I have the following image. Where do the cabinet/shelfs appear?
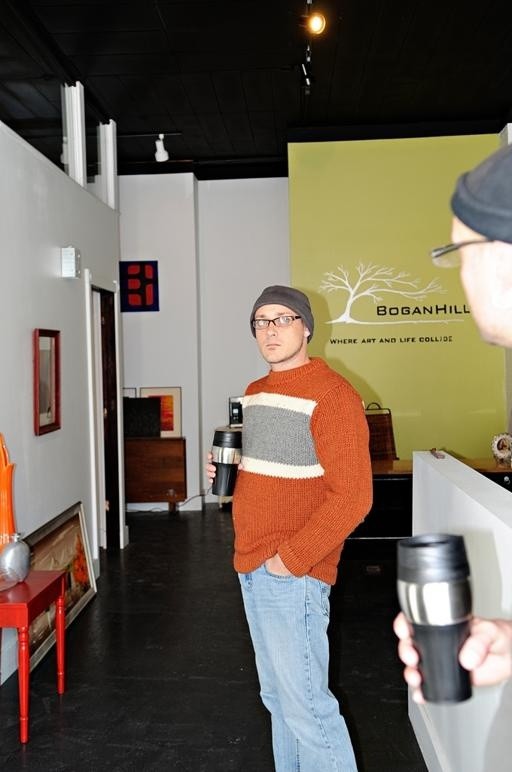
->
[123,438,187,516]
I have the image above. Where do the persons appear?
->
[391,143,511,772]
[204,283,373,772]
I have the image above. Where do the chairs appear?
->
[364,408,400,461]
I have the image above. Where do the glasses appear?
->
[431,238,494,269]
[251,316,302,329]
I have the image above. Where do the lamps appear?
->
[151,134,169,162]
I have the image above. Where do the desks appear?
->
[0,570,66,744]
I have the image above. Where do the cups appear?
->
[395,531,477,705]
[210,429,243,497]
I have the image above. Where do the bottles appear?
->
[1,532,32,583]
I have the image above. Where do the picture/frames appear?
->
[121,387,182,440]
[14,501,98,678]
[32,328,61,437]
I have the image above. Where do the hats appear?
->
[450,141,512,244]
[250,285,314,343]
